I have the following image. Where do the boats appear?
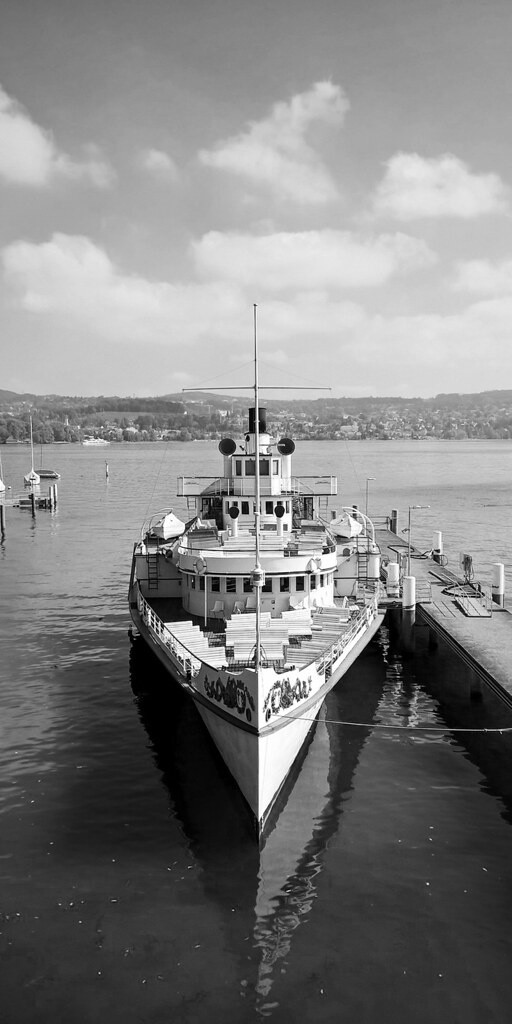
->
[329,511,362,539]
[153,512,186,541]
[129,304,388,847]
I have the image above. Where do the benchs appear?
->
[187,528,327,557]
[162,607,351,675]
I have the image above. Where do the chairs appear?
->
[209,600,226,619]
[232,600,245,614]
[314,598,324,614]
[244,595,257,613]
[288,596,303,611]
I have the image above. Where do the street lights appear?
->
[408,505,432,576]
[366,478,375,516]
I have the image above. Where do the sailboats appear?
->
[0,457,5,492]
[35,429,60,478]
[23,414,41,485]
